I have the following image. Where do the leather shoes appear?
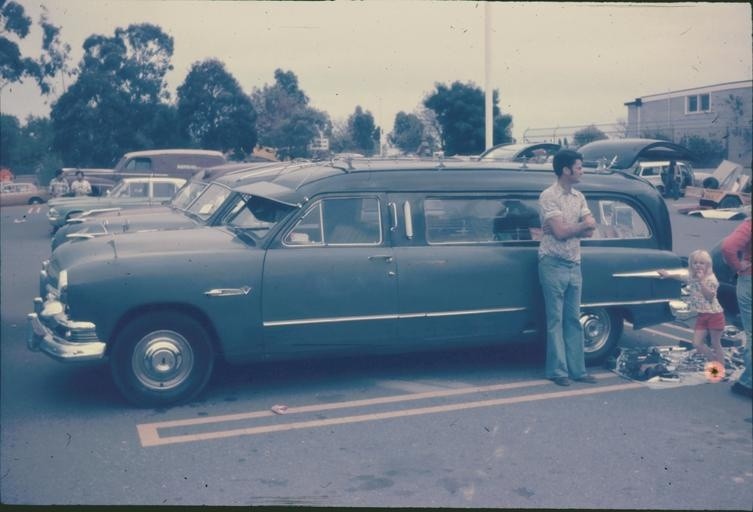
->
[576,375,598,383]
[554,377,569,386]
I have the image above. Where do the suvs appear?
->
[53,150,229,187]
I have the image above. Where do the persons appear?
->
[658,250,725,384]
[71,171,92,198]
[664,160,681,199]
[722,214,753,398]
[537,150,598,386]
[48,169,71,197]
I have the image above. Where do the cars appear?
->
[1,180,48,208]
[33,142,568,257]
[635,161,694,194]
[25,137,698,409]
